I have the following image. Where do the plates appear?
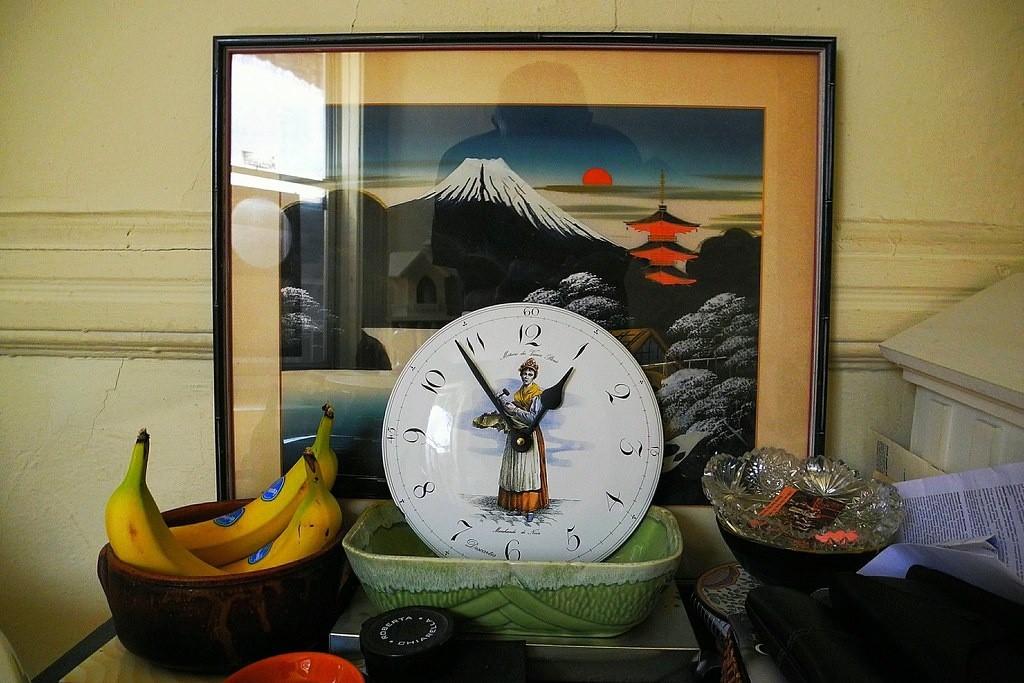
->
[225,650,365,683]
[704,448,903,550]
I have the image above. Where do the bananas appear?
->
[164,402,337,567]
[219,447,342,572]
[104,427,230,576]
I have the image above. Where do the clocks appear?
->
[381,303,665,565]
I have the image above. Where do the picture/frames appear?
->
[212,32,837,506]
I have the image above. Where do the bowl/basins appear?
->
[97,498,343,674]
[716,511,894,597]
